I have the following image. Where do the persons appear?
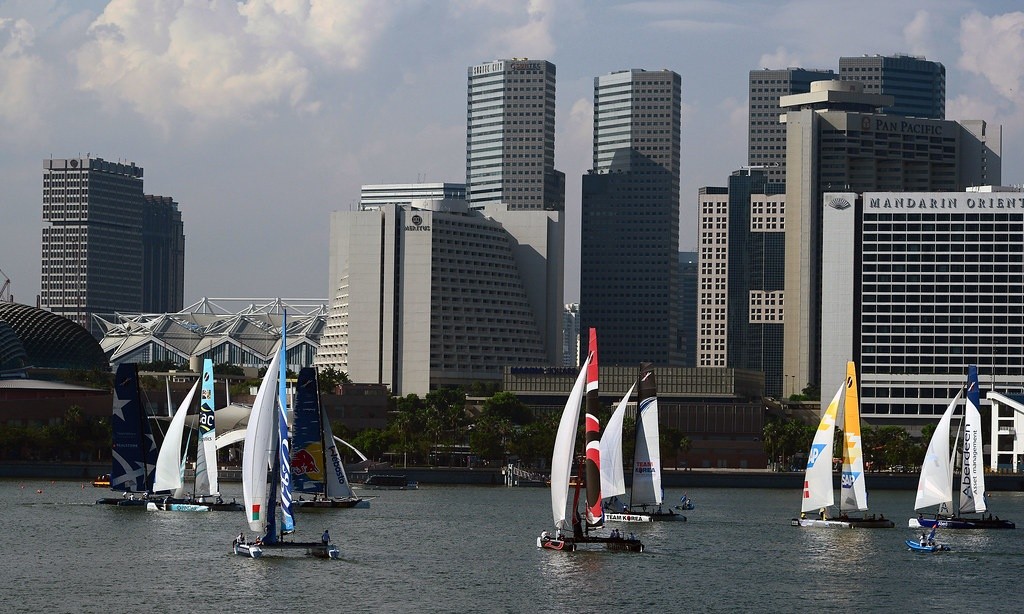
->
[611,529,634,540]
[123,491,149,499]
[541,530,550,541]
[199,495,205,503]
[871,513,884,520]
[236,533,262,545]
[686,498,691,506]
[989,513,999,520]
[603,502,608,510]
[217,496,223,504]
[920,534,927,547]
[321,530,330,546]
[657,507,673,516]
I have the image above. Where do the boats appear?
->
[361,474,420,491]
[673,502,695,511]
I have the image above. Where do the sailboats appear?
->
[536,328,646,555]
[579,361,687,523]
[232,308,341,562]
[904,514,952,553]
[813,361,896,529]
[91,352,374,513]
[908,364,1017,530]
[790,380,856,530]
